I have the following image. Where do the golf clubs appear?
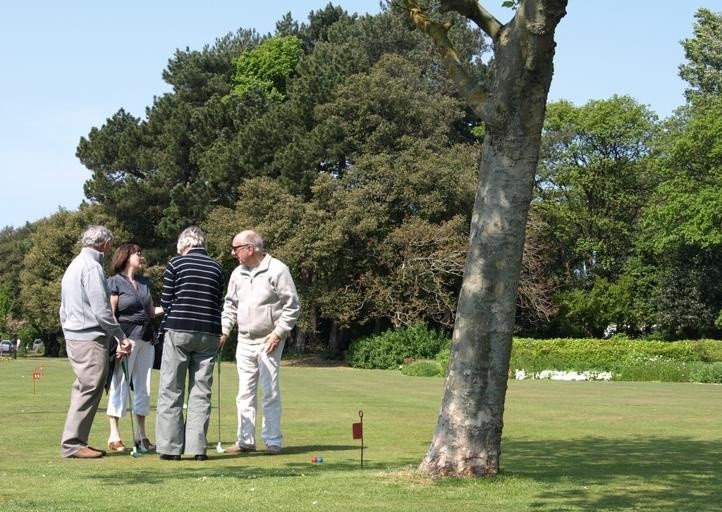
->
[121,353,149,458]
[216,351,224,454]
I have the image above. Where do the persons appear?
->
[58,224,133,458]
[217,229,302,454]
[155,224,227,463]
[99,239,164,455]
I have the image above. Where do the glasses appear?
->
[230,243,255,253]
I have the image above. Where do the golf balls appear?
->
[312,457,317,462]
[317,457,323,462]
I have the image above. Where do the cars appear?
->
[33,338,45,352]
[1,339,15,353]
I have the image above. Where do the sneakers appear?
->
[223,443,256,453]
[133,437,156,451]
[195,454,208,461]
[108,439,125,451]
[264,445,282,454]
[71,445,107,458]
[159,454,181,461]
